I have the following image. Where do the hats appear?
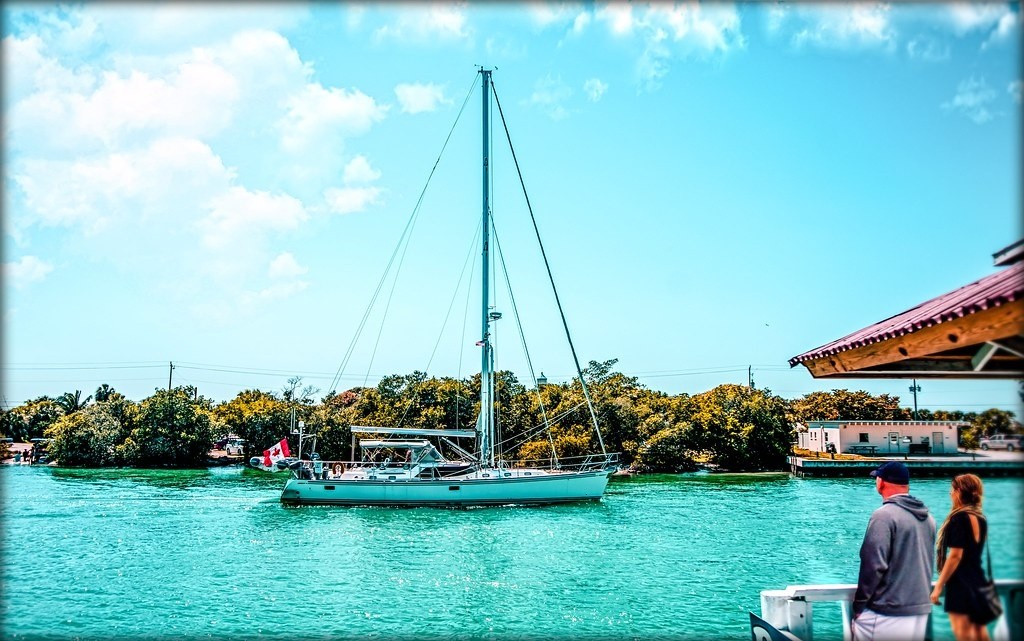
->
[870,461,909,484]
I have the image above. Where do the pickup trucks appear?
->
[980,434,1021,451]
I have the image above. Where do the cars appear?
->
[226,439,255,456]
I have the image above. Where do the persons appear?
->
[620,460,646,474]
[929,473,1003,641]
[15,444,36,461]
[303,458,329,480]
[825,442,835,453]
[851,461,938,641]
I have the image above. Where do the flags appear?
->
[263,438,290,466]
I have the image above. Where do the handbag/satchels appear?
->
[967,581,1004,625]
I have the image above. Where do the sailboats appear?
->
[279,63,620,509]
